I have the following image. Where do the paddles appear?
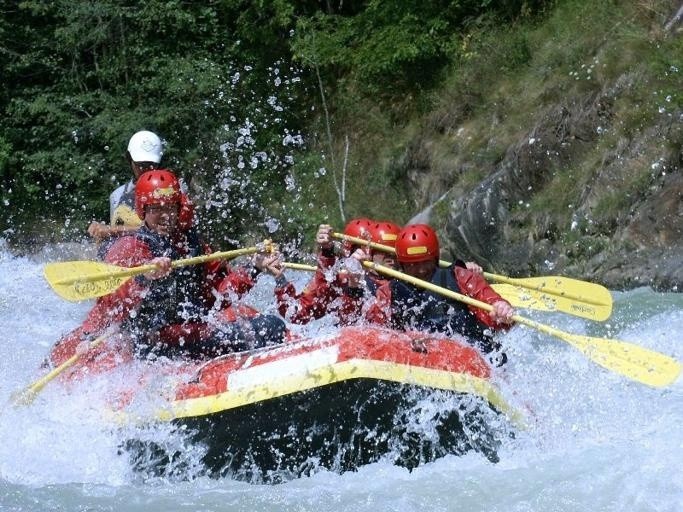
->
[331,230,615,322]
[45,239,274,298]
[360,260,682,390]
[281,259,557,311]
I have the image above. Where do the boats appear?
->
[67,327,518,484]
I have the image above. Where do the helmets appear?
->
[373,217,400,253]
[396,223,442,263]
[134,170,182,221]
[342,219,382,252]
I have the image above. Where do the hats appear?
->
[126,129,164,164]
